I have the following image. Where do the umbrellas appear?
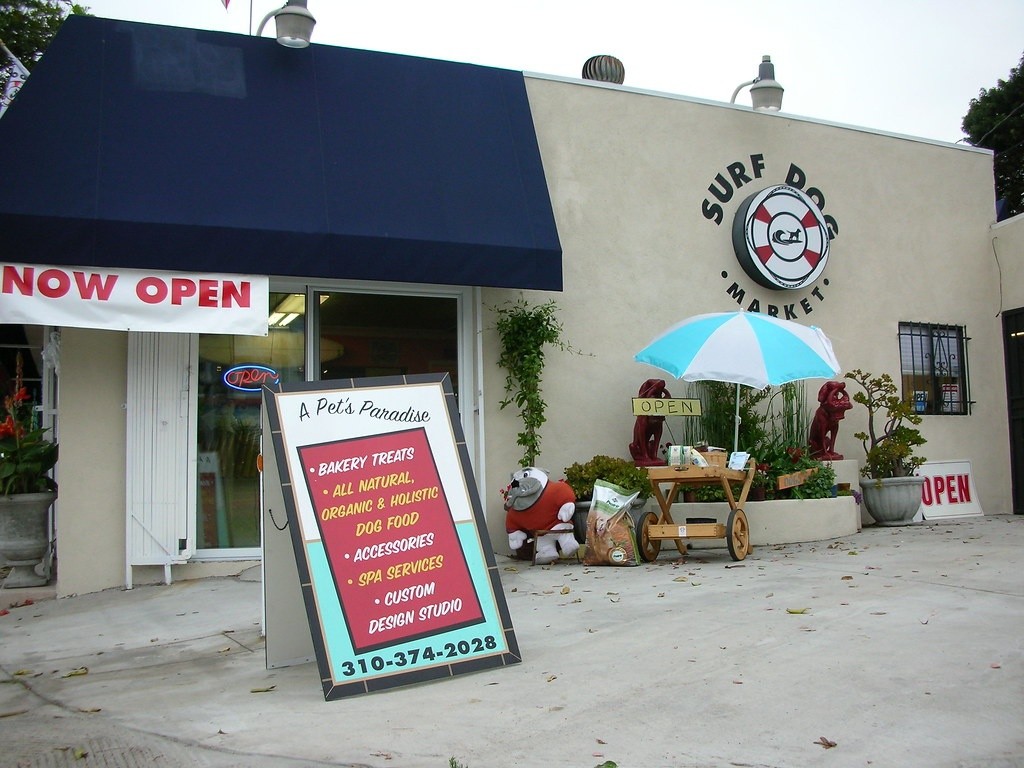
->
[633,307,841,451]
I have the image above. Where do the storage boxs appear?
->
[668,444,693,466]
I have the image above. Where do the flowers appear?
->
[0,387,58,497]
[677,381,863,505]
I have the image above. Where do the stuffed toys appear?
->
[505,467,580,562]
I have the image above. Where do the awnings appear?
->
[0,14,564,293]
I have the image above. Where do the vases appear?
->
[0,492,57,560]
[830,485,837,498]
[685,491,697,502]
[753,487,765,500]
[838,482,850,492]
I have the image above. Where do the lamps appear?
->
[268,292,329,327]
[257,0,317,48]
[730,55,784,112]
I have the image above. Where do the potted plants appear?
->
[563,455,656,546]
[845,369,928,526]
[474,292,598,559]
